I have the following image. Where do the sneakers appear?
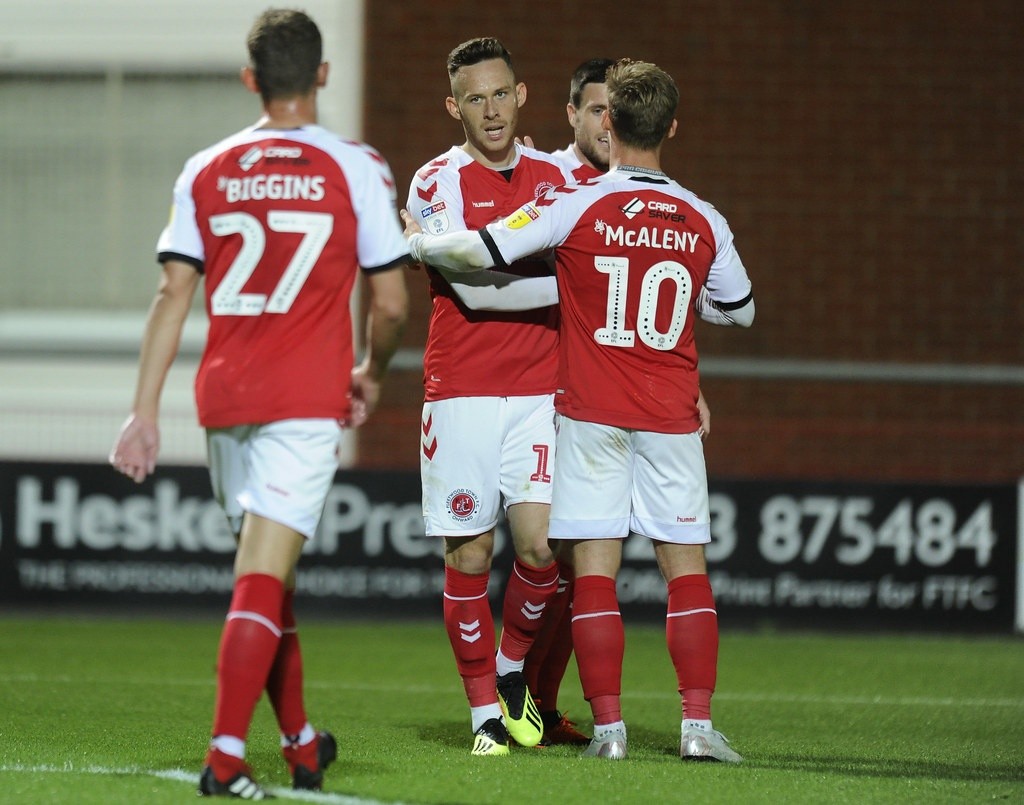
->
[282,731,336,792]
[200,766,278,801]
[496,650,544,746]
[680,721,744,763]
[470,715,510,756]
[581,720,628,760]
[543,711,594,747]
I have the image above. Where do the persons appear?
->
[399,58,756,762]
[407,36,585,754]
[512,60,624,746]
[109,8,413,800]
